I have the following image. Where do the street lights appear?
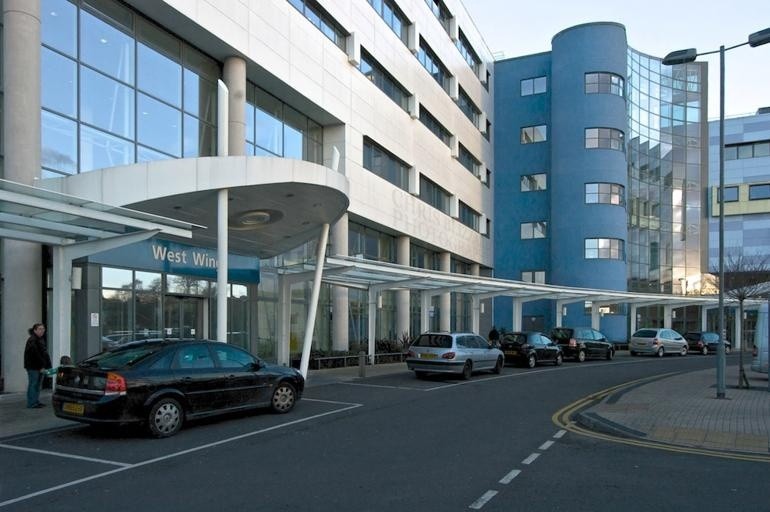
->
[662,27,770,399]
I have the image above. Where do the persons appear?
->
[499,331,505,344]
[23,323,53,408]
[39,356,75,375]
[551,328,567,344]
[489,325,500,347]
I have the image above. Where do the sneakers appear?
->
[33,403,46,408]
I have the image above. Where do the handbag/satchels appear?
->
[39,376,52,390]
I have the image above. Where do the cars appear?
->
[550,326,616,363]
[53,337,303,440]
[405,329,505,380]
[684,332,731,355]
[628,327,689,358]
[499,332,564,368]
